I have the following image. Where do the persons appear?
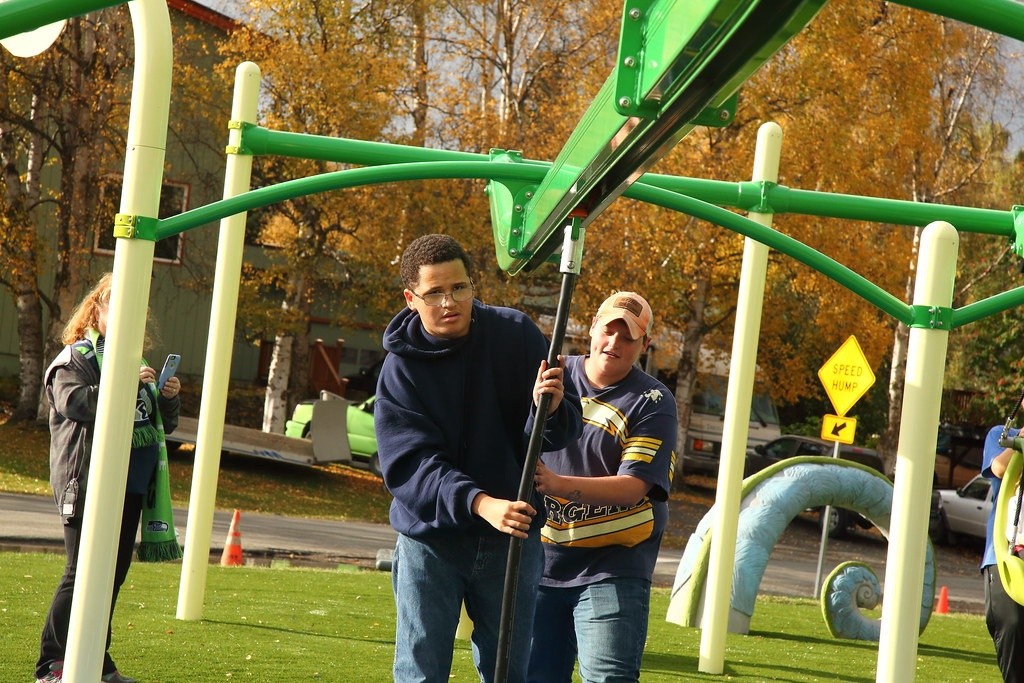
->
[979,387,1024,683]
[533,292,679,683]
[35,270,181,683]
[374,234,584,683]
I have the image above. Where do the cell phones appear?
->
[156,354,181,389]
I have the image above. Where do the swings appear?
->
[993,388,1023,608]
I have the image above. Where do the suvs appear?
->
[662,369,783,475]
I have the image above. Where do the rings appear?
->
[172,389,175,394]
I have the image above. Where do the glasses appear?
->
[407,273,475,306]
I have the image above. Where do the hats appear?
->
[597,292,653,340]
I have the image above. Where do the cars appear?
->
[939,471,997,538]
[285,397,382,480]
[744,435,887,539]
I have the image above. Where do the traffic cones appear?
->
[934,585,951,617]
[219,507,245,568]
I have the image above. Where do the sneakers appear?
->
[35,662,61,683]
[102,672,132,683]
